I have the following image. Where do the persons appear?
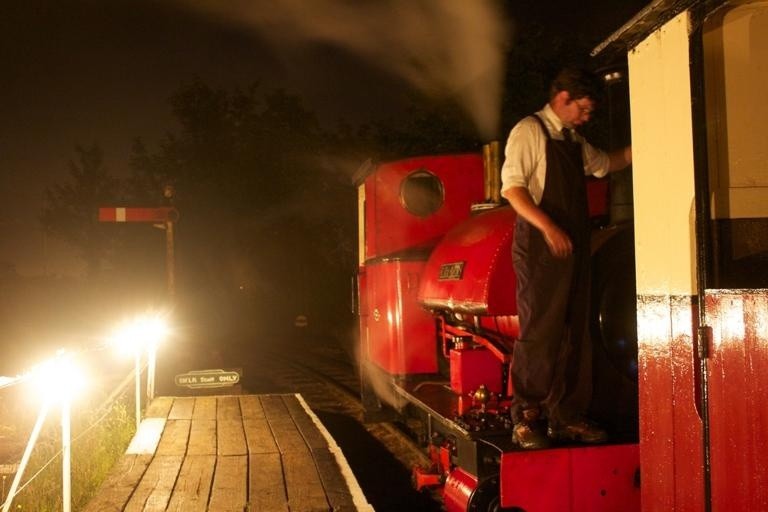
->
[501,67,632,452]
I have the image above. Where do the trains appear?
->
[353,0,768,511]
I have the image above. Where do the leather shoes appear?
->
[512,423,547,449]
[547,422,607,442]
